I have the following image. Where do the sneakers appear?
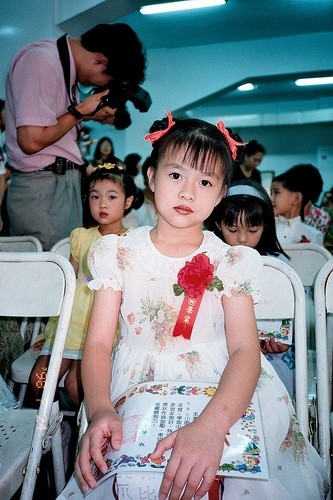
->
[61,421,71,473]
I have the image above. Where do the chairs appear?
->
[0,236,333,500]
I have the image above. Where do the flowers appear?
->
[172,251,224,340]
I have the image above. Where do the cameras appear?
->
[94,79,152,131]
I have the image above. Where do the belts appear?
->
[6,161,79,173]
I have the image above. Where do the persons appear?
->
[0,99,333,500]
[6,22,148,409]
